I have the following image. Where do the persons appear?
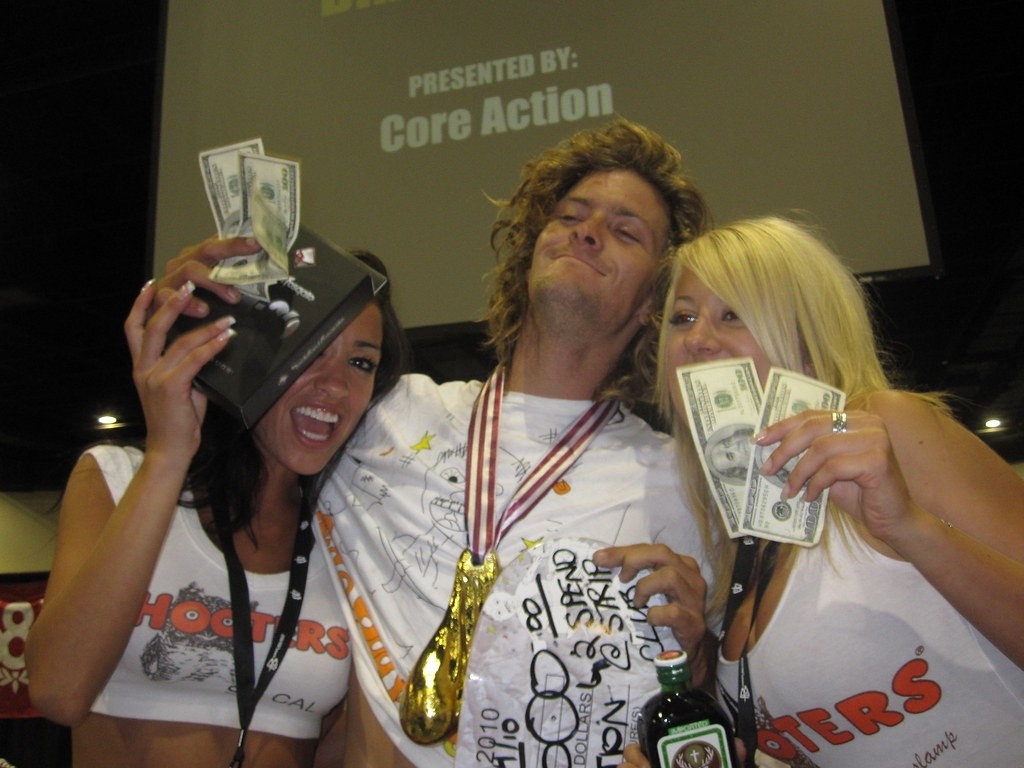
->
[24,124,720,768]
[620,218,1024,768]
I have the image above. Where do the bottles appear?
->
[637,649,742,768]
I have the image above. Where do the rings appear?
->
[830,411,850,437]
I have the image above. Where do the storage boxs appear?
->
[160,224,388,431]
